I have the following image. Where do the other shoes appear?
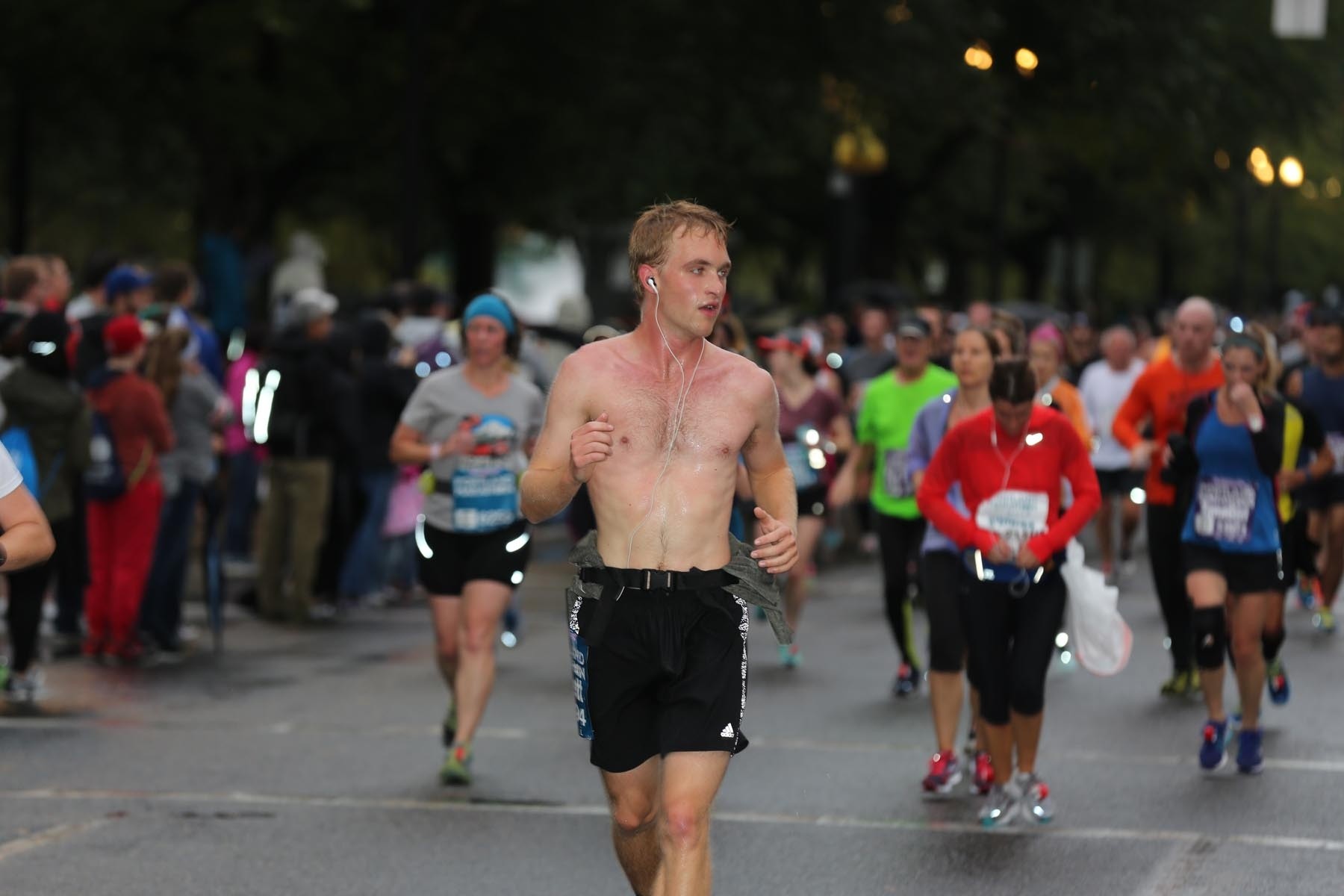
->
[1318,606,1335,635]
[1294,574,1322,609]
[1160,668,1201,694]
[1198,714,1232,771]
[1267,659,1290,705]
[778,641,802,666]
[85,639,146,665]
[1236,726,1266,774]
[978,772,1054,827]
[440,743,472,786]
[923,750,963,793]
[893,662,919,696]
[967,749,996,795]
[442,704,457,744]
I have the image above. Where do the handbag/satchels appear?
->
[1058,537,1131,677]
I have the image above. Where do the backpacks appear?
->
[85,384,153,500]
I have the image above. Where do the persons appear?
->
[518,201,799,896]
[706,294,1344,827]
[0,249,592,786]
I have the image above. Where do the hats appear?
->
[896,313,932,337]
[362,322,402,349]
[756,329,821,357]
[104,264,157,302]
[285,286,339,322]
[105,313,159,358]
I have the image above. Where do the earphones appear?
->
[649,277,660,294]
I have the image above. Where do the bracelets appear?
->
[430,443,442,459]
